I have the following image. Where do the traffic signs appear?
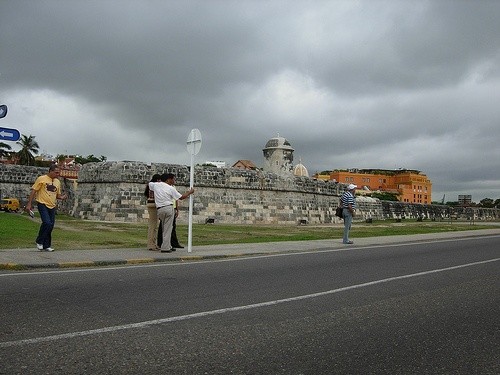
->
[1,127,20,142]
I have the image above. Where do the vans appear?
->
[0,198,20,213]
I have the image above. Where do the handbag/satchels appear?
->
[335,207,343,218]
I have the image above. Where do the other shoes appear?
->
[45,247,55,252]
[344,240,353,244]
[161,249,172,253]
[173,245,184,249]
[36,242,43,250]
[171,249,176,251]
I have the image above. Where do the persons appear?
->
[27,165,68,252]
[143,172,195,254]
[339,183,357,244]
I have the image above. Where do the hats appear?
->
[348,184,357,191]
